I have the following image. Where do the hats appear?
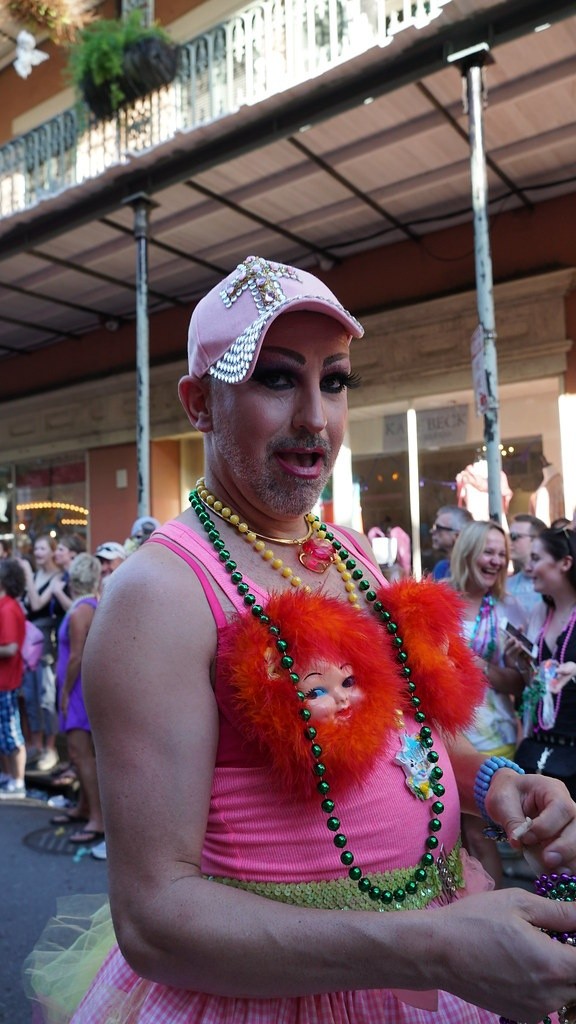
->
[96,542,125,560]
[187,255,365,387]
[131,516,161,536]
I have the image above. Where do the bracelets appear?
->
[476,755,526,842]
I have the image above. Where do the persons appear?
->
[36,255,575,1024]
[0,517,157,861]
[431,504,575,802]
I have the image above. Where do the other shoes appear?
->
[90,841,108,858]
[0,782,25,801]
[36,748,59,770]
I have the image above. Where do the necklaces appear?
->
[196,477,360,612]
[198,486,336,573]
[472,597,498,665]
[187,468,446,902]
[538,608,575,729]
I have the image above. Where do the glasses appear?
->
[433,524,455,532]
[509,532,531,541]
[556,524,572,546]
[96,545,120,552]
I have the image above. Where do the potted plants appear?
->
[62,9,179,120]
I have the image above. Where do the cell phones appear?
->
[498,617,539,659]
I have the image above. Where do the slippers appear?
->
[49,813,87,825]
[69,830,104,844]
